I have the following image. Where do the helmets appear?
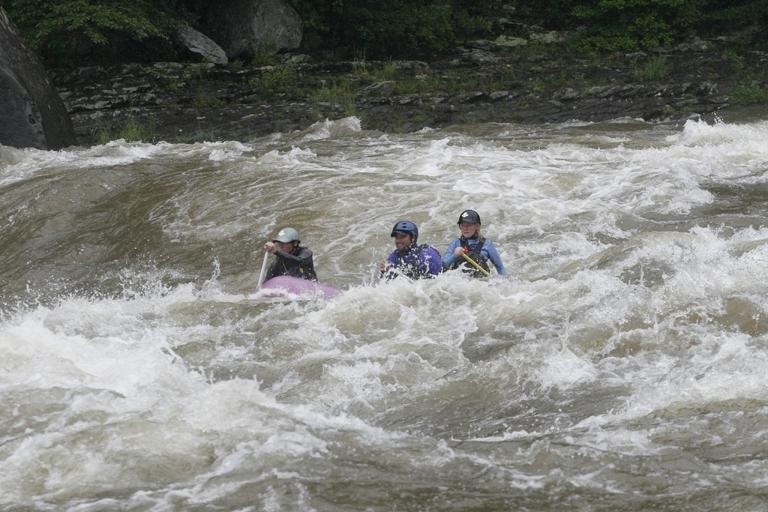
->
[273,227,300,245]
[457,210,481,224]
[391,220,418,242]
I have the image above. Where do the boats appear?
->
[264,275,346,302]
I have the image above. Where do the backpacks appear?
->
[457,236,491,279]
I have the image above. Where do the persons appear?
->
[262,226,317,286]
[442,209,508,282]
[377,219,442,279]
